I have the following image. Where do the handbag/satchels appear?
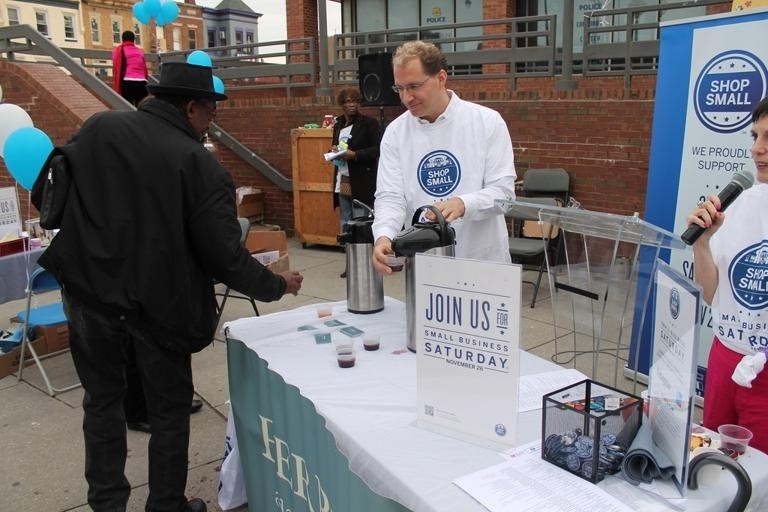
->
[40,155,72,229]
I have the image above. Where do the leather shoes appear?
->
[127,420,151,433]
[187,498,207,512]
[189,400,202,412]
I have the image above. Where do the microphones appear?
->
[680,170,755,246]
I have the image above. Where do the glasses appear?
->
[201,104,217,118]
[391,77,430,93]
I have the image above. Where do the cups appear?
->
[336,349,355,368]
[387,254,405,272]
[361,332,379,351]
[324,114,333,129]
[716,424,753,462]
[316,304,333,320]
[334,339,353,356]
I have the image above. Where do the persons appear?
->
[35,58,305,510]
[327,85,382,277]
[689,98,767,452]
[368,40,517,278]
[112,30,152,107]
[122,95,204,436]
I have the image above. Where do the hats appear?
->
[146,62,227,101]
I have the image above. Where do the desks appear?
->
[223,295,768,512]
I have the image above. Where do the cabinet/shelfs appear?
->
[289,126,346,251]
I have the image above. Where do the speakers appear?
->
[358,52,403,107]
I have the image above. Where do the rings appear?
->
[696,200,704,209]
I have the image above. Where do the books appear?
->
[320,150,347,162]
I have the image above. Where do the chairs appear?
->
[214,218,260,335]
[505,167,572,308]
[16,265,82,397]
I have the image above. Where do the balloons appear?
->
[209,75,225,95]
[186,51,213,68]
[3,126,55,190]
[133,2,151,27]
[144,0,162,18]
[0,104,35,158]
[155,0,180,27]
